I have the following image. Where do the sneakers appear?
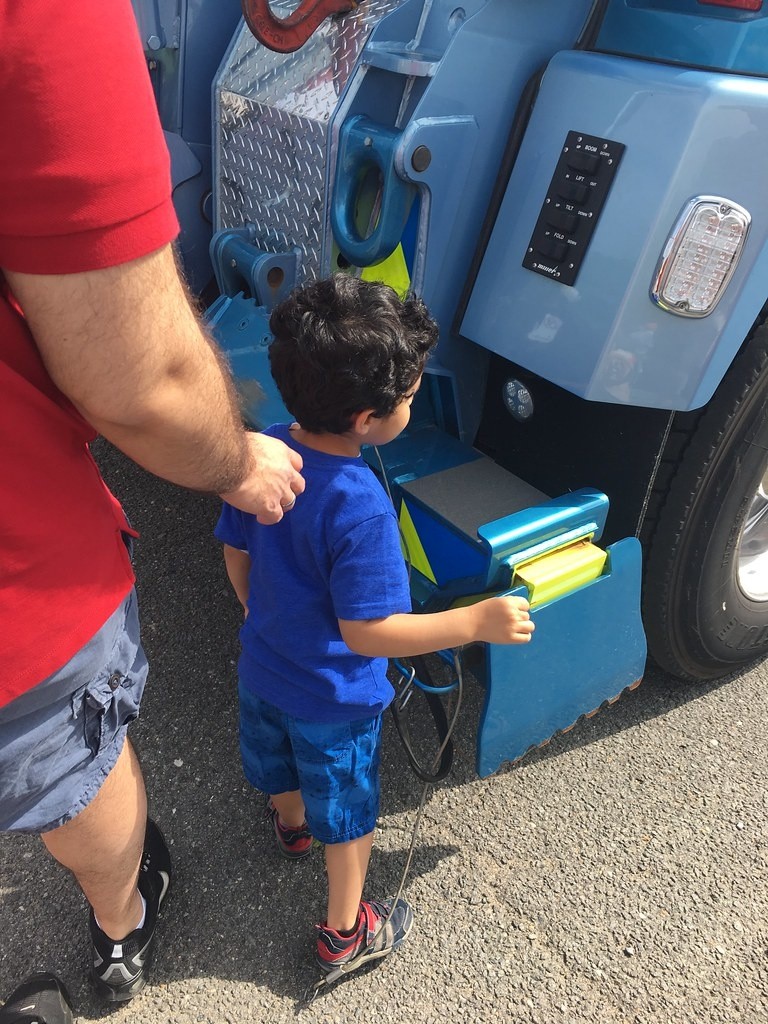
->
[89,816,171,1001]
[315,899,414,972]
[263,797,313,859]
[0,970,73,1024]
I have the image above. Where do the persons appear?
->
[215,274,535,995]
[0,3,310,1004]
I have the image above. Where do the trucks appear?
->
[125,0,768,780]
